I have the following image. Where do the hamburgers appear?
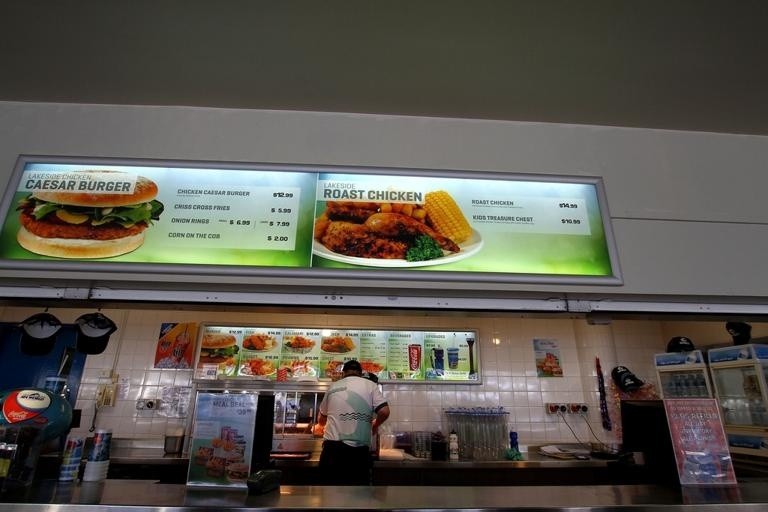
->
[226,463,249,483]
[225,453,243,470]
[552,368,562,377]
[200,334,239,366]
[16,170,164,259]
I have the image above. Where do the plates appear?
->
[243,341,277,352]
[321,347,356,355]
[286,367,317,379]
[240,364,276,377]
[314,219,484,268]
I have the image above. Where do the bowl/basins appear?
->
[285,345,313,354]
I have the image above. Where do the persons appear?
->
[317,359,390,486]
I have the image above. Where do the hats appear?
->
[342,360,362,371]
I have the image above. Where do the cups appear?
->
[45,376,66,395]
[447,348,459,369]
[171,334,191,364]
[408,345,421,371]
[221,426,245,455]
[57,429,113,482]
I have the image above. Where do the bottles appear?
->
[434,345,444,370]
[509,428,519,451]
[664,374,704,398]
[449,430,459,461]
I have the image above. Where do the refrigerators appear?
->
[653,342,768,459]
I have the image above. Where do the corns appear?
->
[423,191,472,245]
